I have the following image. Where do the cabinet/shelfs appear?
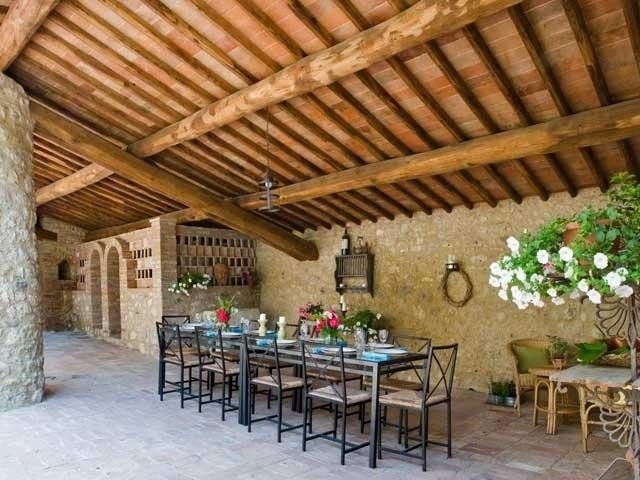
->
[334,253,375,298]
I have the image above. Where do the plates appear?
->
[374,349,408,355]
[323,347,357,353]
[367,343,395,349]
[221,333,241,336]
[254,329,275,334]
[276,340,298,344]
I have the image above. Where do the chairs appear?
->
[360,335,432,444]
[156,321,213,408]
[248,323,298,409]
[194,325,255,421]
[506,337,568,418]
[299,339,373,465]
[161,315,209,394]
[243,333,313,443]
[305,369,362,429]
[377,343,458,473]
[210,320,260,391]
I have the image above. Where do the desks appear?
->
[549,363,640,454]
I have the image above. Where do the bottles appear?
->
[341,228,350,256]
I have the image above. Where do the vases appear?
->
[353,327,368,360]
[562,219,622,264]
[175,288,204,323]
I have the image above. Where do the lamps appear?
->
[257,169,280,213]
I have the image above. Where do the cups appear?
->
[379,330,388,349]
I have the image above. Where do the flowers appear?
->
[167,270,211,296]
[337,309,381,339]
[488,171,639,310]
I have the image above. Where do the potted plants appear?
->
[504,380,517,407]
[545,334,569,369]
